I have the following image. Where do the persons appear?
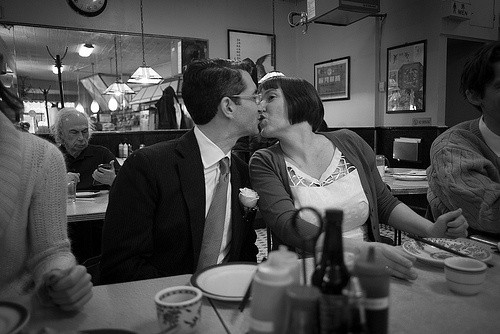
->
[95,59,261,285]
[426,42,500,232]
[51,107,122,264]
[249,76,468,279]
[0,36,94,334]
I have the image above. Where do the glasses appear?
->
[231,91,263,105]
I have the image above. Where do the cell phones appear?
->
[93,164,112,185]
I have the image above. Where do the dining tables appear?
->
[14,237,500,334]
[381,166,429,244]
[64,190,109,221]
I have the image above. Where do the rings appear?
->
[386,264,390,270]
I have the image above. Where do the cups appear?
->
[442,256,487,296]
[376,155,385,178]
[64,176,77,203]
[155,286,203,334]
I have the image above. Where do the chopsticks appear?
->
[405,233,495,269]
[238,257,269,311]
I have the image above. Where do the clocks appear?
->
[66,0,107,17]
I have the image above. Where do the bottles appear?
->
[118,143,132,158]
[249,207,391,334]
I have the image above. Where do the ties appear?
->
[194,158,230,273]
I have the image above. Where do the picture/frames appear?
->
[386,40,425,113]
[313,56,351,101]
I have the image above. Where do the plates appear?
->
[393,172,428,181]
[189,261,264,302]
[401,238,492,267]
[76,191,100,199]
[0,301,31,334]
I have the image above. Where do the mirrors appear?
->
[0,19,209,137]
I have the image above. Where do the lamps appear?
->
[127,0,163,85]
[50,32,133,113]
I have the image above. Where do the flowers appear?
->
[239,186,260,222]
